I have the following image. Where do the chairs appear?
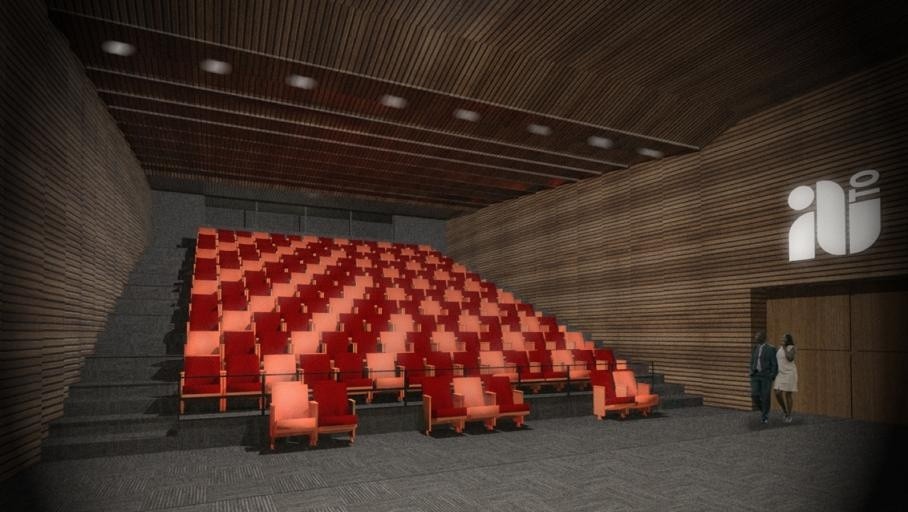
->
[180,224,659,452]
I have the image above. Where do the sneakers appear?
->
[761,416,768,423]
[784,415,792,424]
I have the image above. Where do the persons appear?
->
[772,332,799,426]
[746,330,778,423]
